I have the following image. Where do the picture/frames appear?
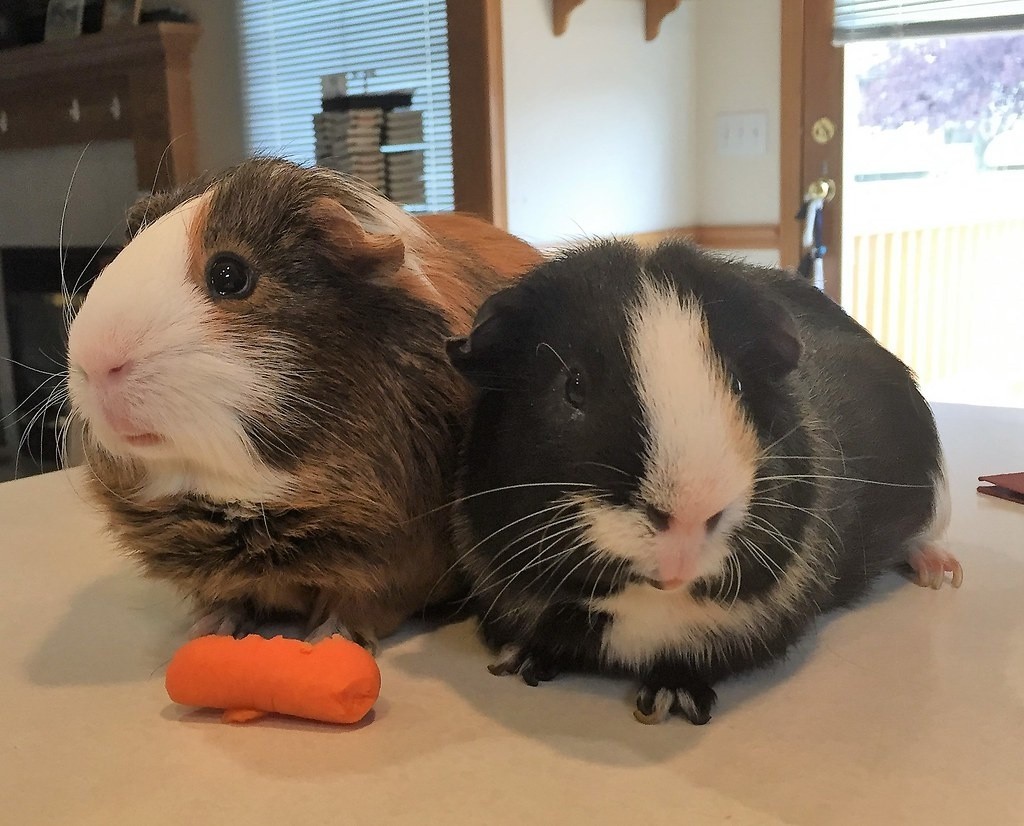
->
[101,1,141,30]
[43,0,85,42]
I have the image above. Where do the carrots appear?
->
[166,633,381,723]
